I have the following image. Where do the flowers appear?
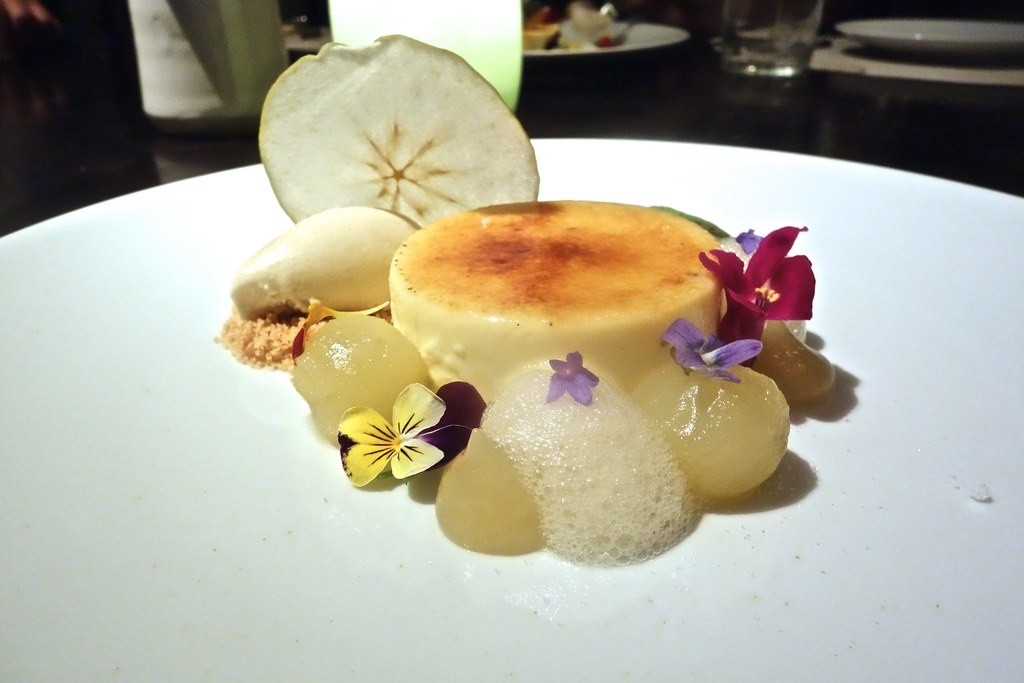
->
[699,225,815,350]
[660,319,764,382]
[337,381,486,488]
[546,352,599,405]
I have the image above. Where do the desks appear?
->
[0,0,1024,238]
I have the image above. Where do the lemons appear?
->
[259,34,538,231]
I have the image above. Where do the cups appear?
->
[127,0,288,132]
[327,0,523,120]
[720,0,827,78]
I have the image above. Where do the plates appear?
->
[282,26,331,52]
[523,23,689,59]
[837,19,1024,56]
[0,138,1024,683]
